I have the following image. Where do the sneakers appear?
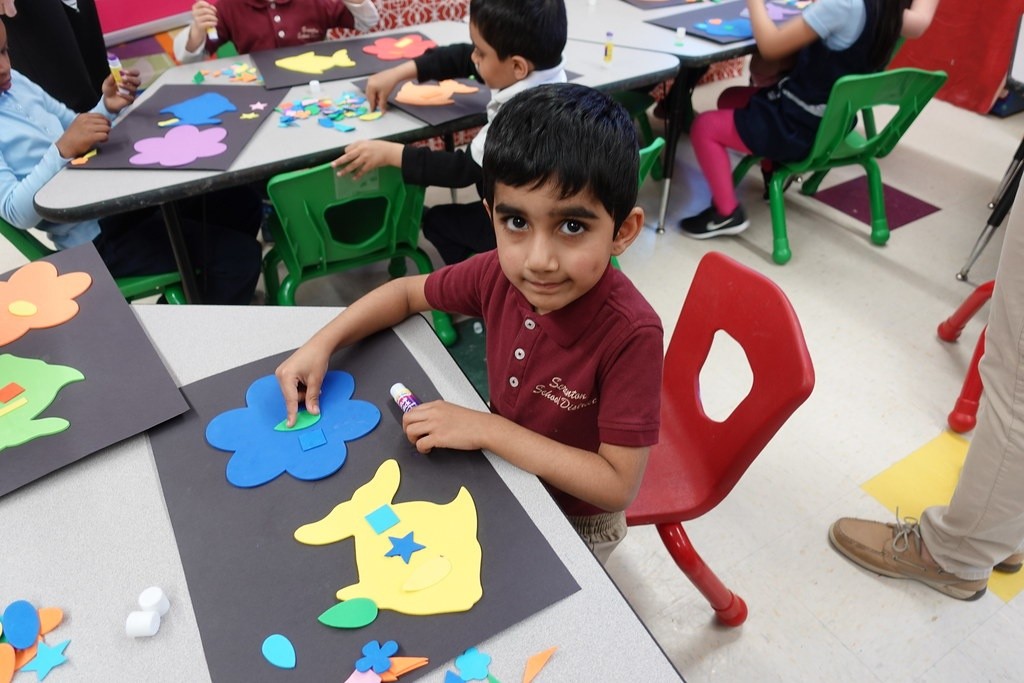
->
[678,206,750,239]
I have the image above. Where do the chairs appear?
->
[731,67,949,263]
[0,218,198,304]
[624,251,814,627]
[609,89,664,271]
[261,156,459,348]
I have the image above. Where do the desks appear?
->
[33,19,680,305]
[2,305,687,683]
[461,1,823,231]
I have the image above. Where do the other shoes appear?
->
[829,518,987,601]
[993,552,1024,574]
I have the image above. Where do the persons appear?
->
[0,18,263,304]
[171,0,379,67]
[272,82,665,577]
[828,167,1023,604]
[651,54,717,140]
[677,0,941,238]
[330,0,571,270]
[0,0,113,113]
